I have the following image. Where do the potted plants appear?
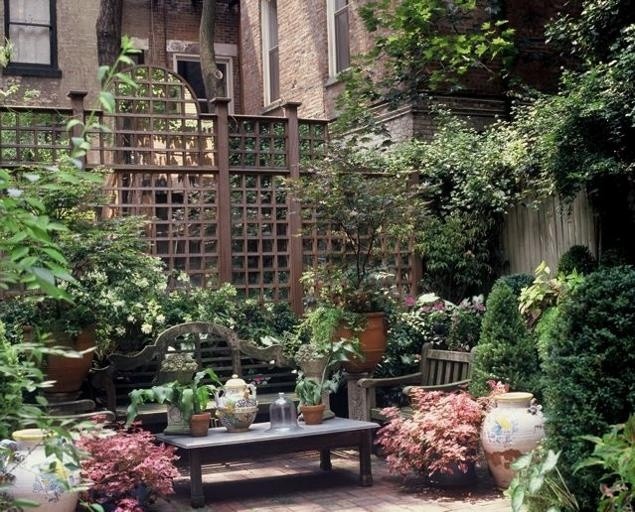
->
[294,338,366,424]
[274,136,441,371]
[124,366,227,437]
[0,149,169,394]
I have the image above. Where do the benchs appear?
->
[89,321,330,437]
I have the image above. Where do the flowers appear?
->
[373,380,511,484]
[71,413,181,511]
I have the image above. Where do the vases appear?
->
[1,428,81,512]
[423,445,470,489]
[480,392,547,492]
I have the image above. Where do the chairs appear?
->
[356,343,472,490]
